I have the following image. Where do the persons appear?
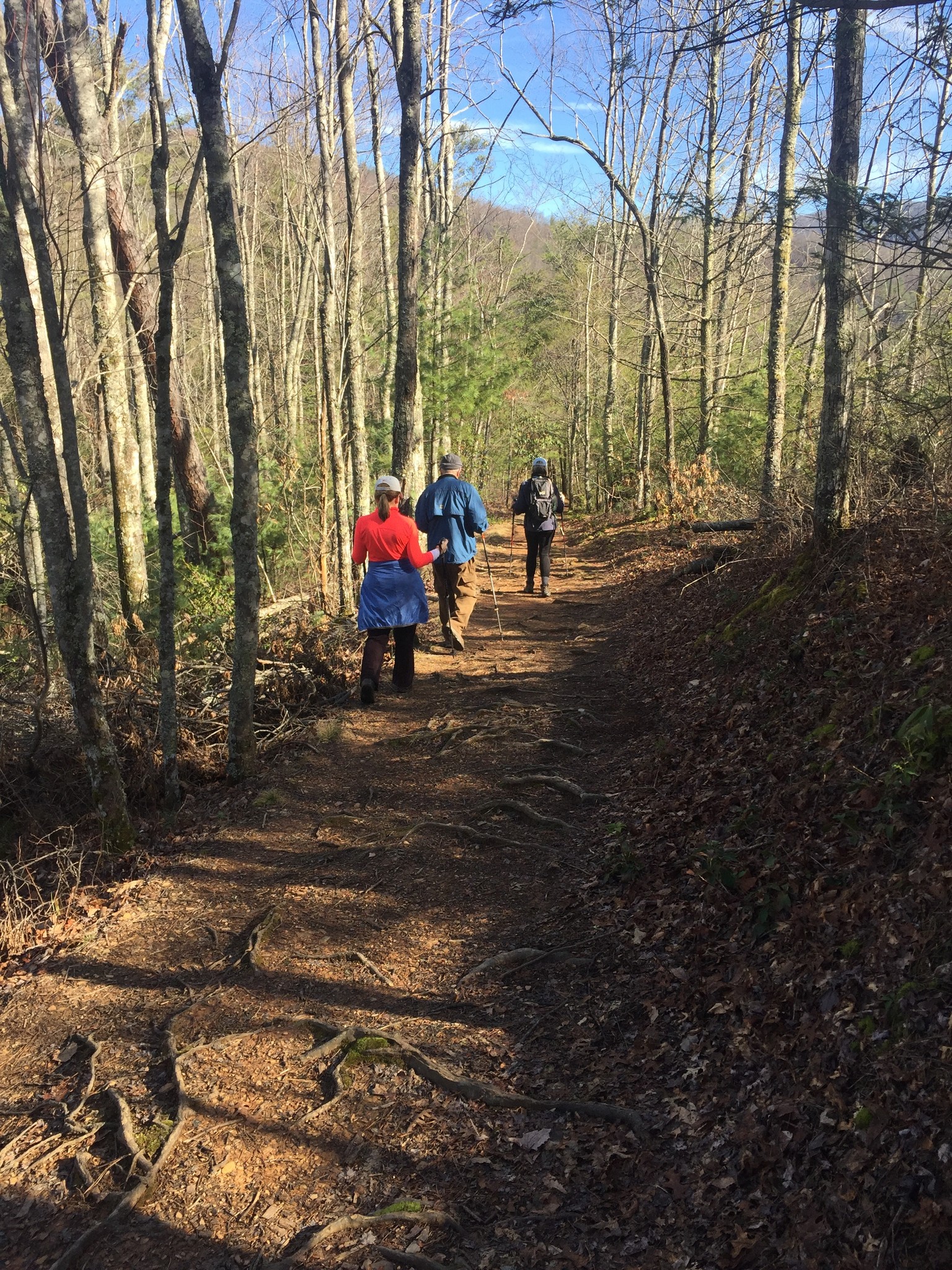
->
[351,476,449,703]
[511,457,565,596]
[416,453,489,650]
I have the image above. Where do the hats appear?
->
[532,457,546,467]
[439,453,463,470]
[374,475,404,499]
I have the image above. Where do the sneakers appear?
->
[444,625,464,651]
[443,640,451,648]
[360,679,374,704]
[396,681,413,692]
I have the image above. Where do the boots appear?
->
[523,581,534,591]
[540,583,550,596]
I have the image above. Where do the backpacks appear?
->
[525,477,555,520]
